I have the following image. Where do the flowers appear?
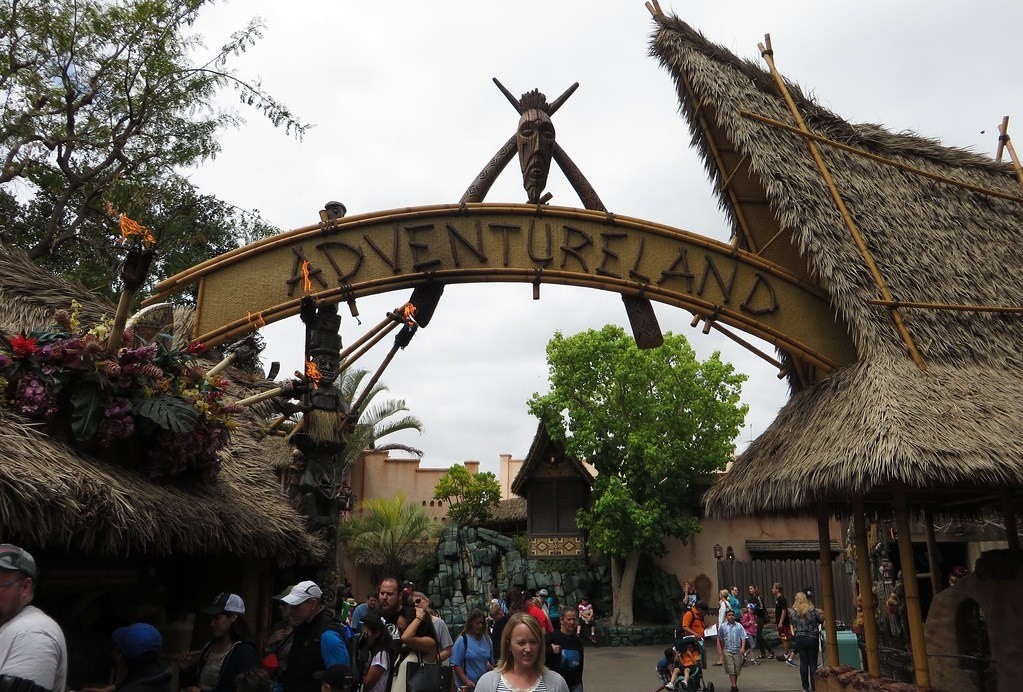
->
[0,303,235,483]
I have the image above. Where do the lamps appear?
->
[727,545,733,559]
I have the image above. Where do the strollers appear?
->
[673,634,714,692]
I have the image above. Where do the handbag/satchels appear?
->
[756,606,770,626]
[406,642,451,692]
[260,654,278,674]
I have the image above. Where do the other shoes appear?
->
[730,685,740,692]
[665,683,675,690]
[588,636,596,643]
[576,633,580,638]
[743,653,748,662]
[776,655,789,661]
[786,660,796,667]
[712,663,723,666]
[681,679,687,689]
[768,649,775,659]
[756,654,767,658]
[750,659,760,665]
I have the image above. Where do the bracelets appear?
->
[416,617,422,621]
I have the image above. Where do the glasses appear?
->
[413,598,424,606]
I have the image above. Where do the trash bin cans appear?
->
[819,630,861,669]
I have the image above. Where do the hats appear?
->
[280,581,323,605]
[720,589,728,597]
[539,589,548,596]
[272,585,295,600]
[747,603,756,610]
[113,623,162,660]
[344,598,357,606]
[0,544,36,578]
[202,592,245,617]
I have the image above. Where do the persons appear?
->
[656,579,711,692]
[715,608,748,692]
[772,583,792,661]
[0,543,454,692]
[450,587,597,692]
[786,592,825,692]
[517,109,556,201]
[286,332,355,444]
[713,582,774,666]
